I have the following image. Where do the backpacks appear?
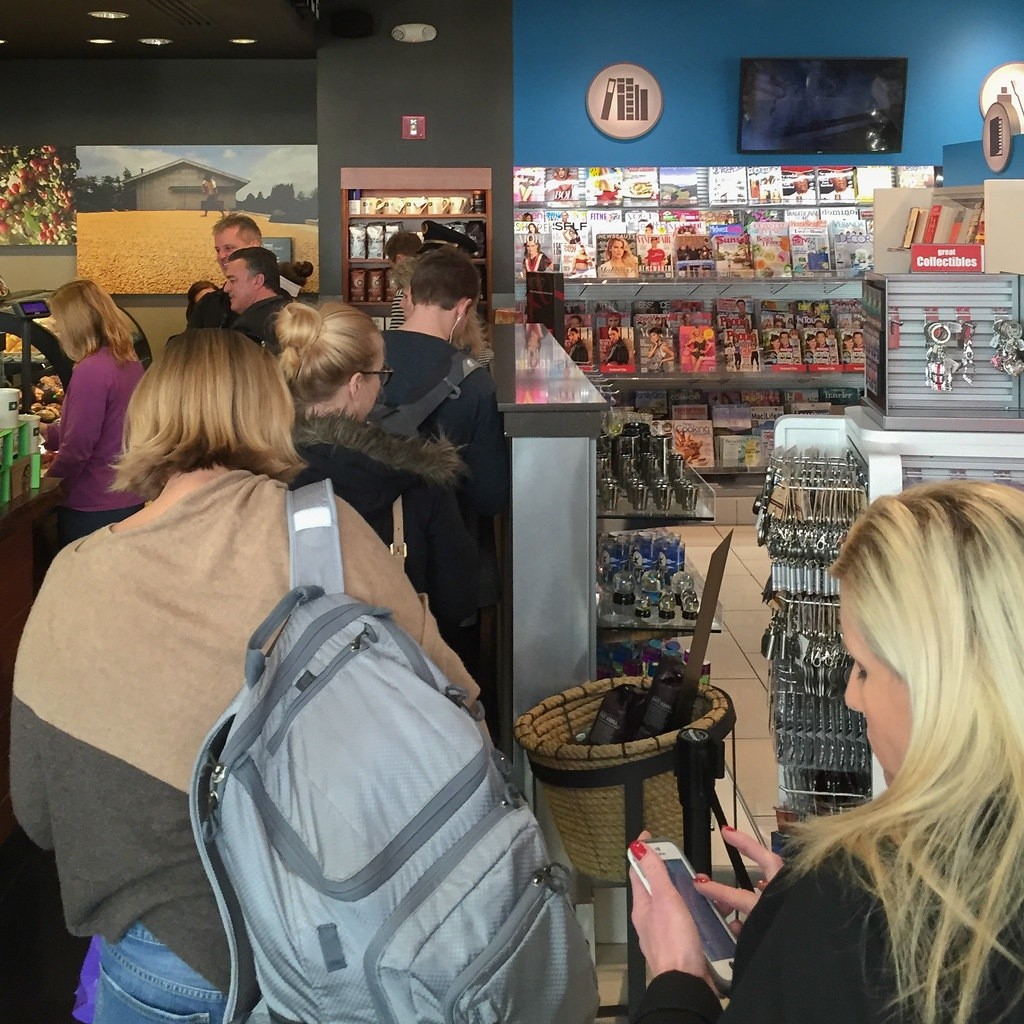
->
[187,476,601,1024]
[364,349,483,443]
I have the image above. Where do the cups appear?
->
[18,414,41,454]
[596,423,699,512]
[0,388,20,429]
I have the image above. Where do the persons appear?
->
[32,279,147,548]
[8,326,492,1024]
[515,163,938,469]
[160,214,505,738]
[622,479,1024,1024]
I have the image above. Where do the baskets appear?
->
[512,675,729,887]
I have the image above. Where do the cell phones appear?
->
[626,837,737,995]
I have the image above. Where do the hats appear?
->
[416,219,481,257]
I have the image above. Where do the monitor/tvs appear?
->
[738,57,908,155]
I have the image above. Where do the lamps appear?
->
[585,60,664,139]
[977,60,1023,134]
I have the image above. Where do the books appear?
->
[904,203,985,246]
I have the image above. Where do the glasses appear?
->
[359,364,394,387]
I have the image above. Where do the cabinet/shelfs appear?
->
[871,176,1024,275]
[339,166,494,353]
[489,322,773,1020]
[861,269,1021,433]
[514,201,872,526]
[1,290,152,423]
[754,404,1023,858]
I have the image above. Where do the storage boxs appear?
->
[516,674,738,888]
